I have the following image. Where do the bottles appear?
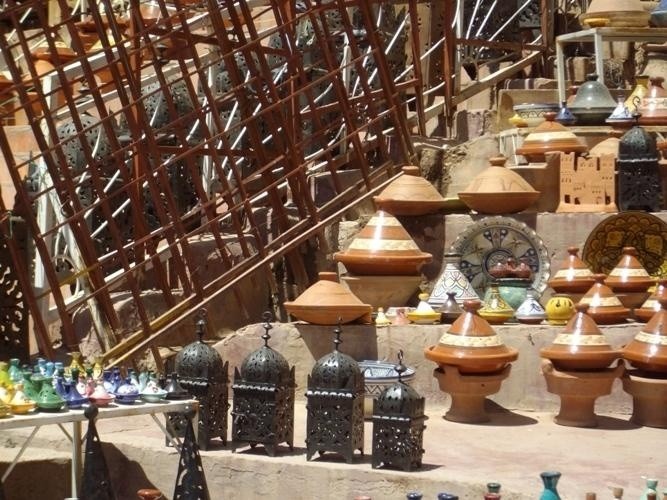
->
[429,300,516,372]
[0,357,188,421]
[457,155,542,215]
[334,197,433,276]
[515,111,587,157]
[540,302,620,371]
[539,469,562,499]
[374,245,667,325]
[555,71,666,125]
[372,164,448,216]
[283,272,371,324]
[623,296,666,373]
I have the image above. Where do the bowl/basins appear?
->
[358,362,415,422]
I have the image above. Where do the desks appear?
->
[0,398,201,500]
[556,25,667,103]
